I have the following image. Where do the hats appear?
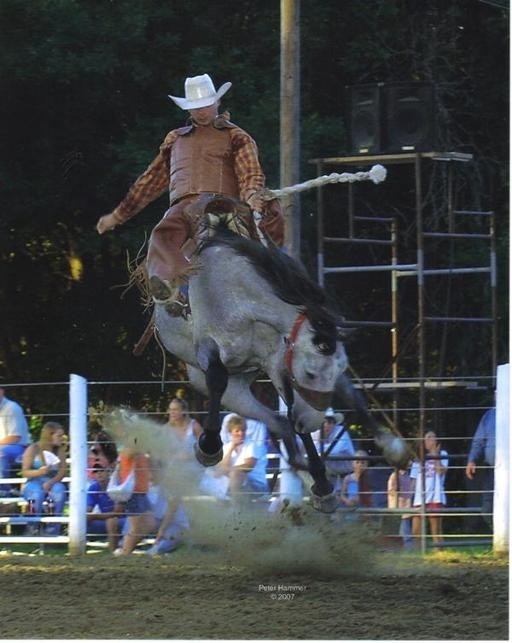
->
[167,73,233,111]
[323,407,344,425]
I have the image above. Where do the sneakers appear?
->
[148,275,186,319]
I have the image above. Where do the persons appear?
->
[1,383,449,555]
[465,387,495,531]
[97,71,284,318]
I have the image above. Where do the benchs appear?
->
[0,452,312,548]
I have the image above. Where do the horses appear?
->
[152,164,413,514]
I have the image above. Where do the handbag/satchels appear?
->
[106,463,135,502]
